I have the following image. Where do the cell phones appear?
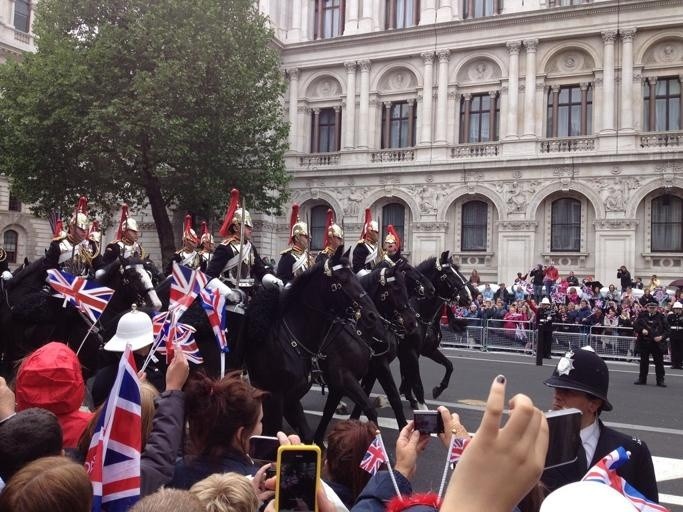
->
[274,444,321,512]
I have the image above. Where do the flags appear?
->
[587,446,669,511]
[450,435,470,464]
[154,323,208,366]
[168,260,209,323]
[84,343,142,511]
[359,437,384,476]
[202,276,235,353]
[148,307,168,343]
[46,267,117,322]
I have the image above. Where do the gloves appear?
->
[262,274,283,289]
[204,277,239,303]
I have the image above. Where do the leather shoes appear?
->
[634,378,646,384]
[657,380,666,386]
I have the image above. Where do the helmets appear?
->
[543,347,614,412]
[672,302,682,308]
[542,297,550,305]
[103,304,155,352]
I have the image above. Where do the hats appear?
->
[647,300,658,307]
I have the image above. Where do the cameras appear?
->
[544,408,584,469]
[250,436,280,463]
[413,409,443,435]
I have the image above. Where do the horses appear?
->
[0,248,166,377]
[314,258,417,441]
[225,244,378,458]
[349,245,434,432]
[419,255,477,399]
[396,250,472,409]
[0,247,76,351]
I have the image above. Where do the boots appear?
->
[224,310,248,370]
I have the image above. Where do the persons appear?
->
[41,214,90,275]
[315,227,344,266]
[101,218,143,262]
[211,209,289,299]
[200,233,214,268]
[381,234,402,266]
[277,217,314,278]
[1,263,683,512]
[345,221,384,271]
[167,229,201,274]
